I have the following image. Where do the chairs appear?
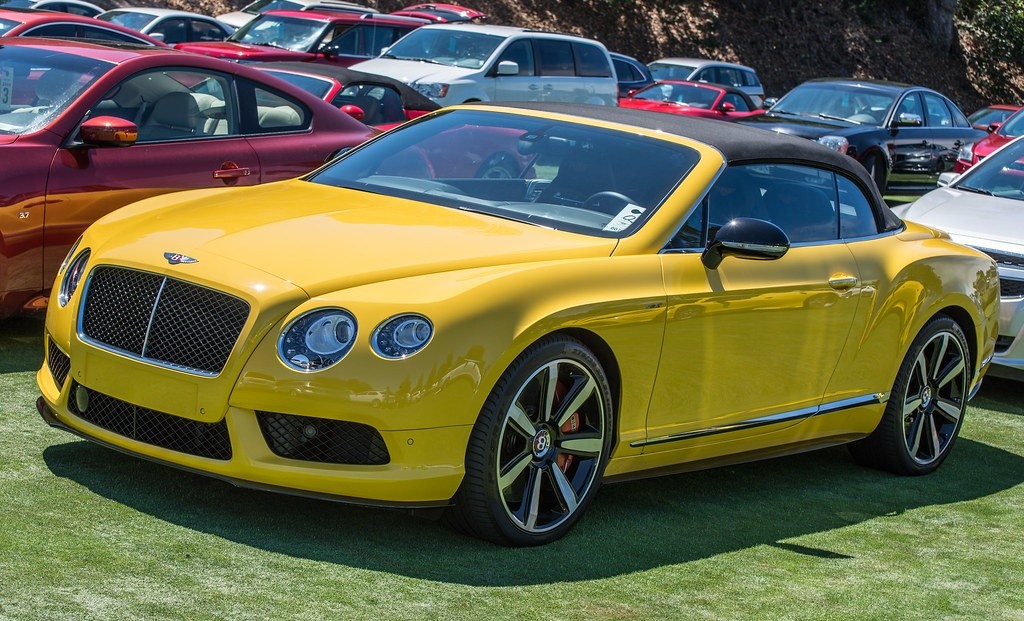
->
[535,153,641,210]
[761,182,834,232]
[882,103,907,119]
[352,96,384,122]
[652,168,769,228]
[11,68,80,116]
[146,92,200,143]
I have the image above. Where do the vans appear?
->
[348,19,618,111]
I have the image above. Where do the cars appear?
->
[0,3,1024,545]
